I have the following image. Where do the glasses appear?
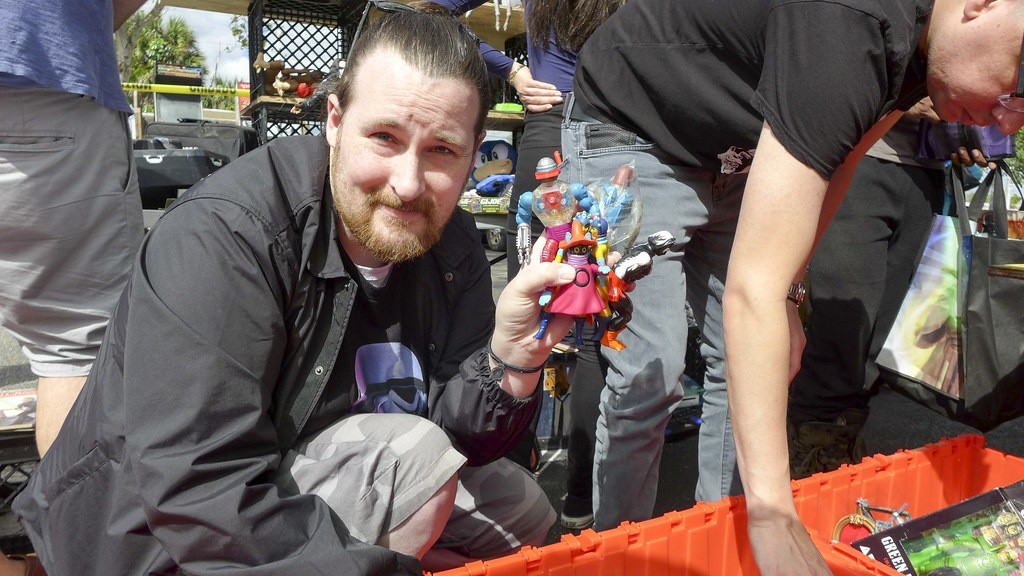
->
[997,33,1024,113]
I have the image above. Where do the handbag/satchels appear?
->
[860,160,1024,424]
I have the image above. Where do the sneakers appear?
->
[560,496,594,529]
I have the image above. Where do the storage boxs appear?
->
[422,434,1024,576]
[248,0,367,147]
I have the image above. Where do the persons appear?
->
[0,0,630,576]
[561,1,1024,576]
[515,151,675,352]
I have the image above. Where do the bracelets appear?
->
[787,282,806,307]
[508,64,526,86]
[488,336,545,383]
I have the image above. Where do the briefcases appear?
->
[142,117,259,172]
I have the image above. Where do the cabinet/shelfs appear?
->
[389,0,524,125]
